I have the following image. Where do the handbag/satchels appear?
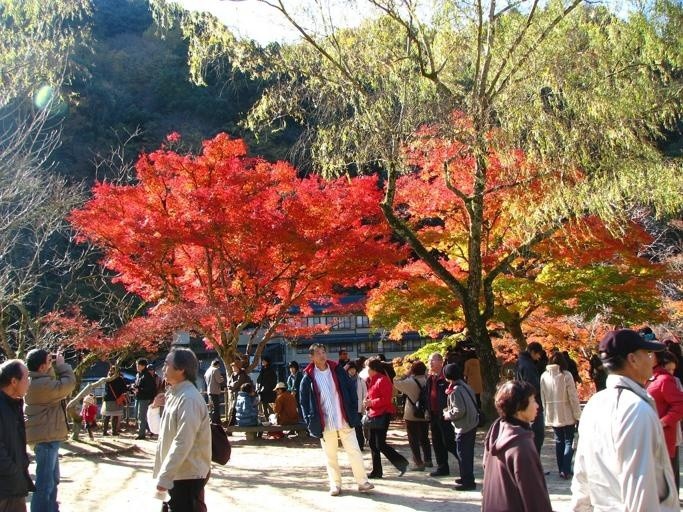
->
[477,411,485,427]
[210,424,230,464]
[117,393,129,406]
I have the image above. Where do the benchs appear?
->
[226,422,308,442]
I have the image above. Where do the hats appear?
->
[443,363,461,380]
[599,330,665,363]
[273,382,286,391]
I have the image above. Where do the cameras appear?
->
[51,354,57,361]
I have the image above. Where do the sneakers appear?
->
[330,486,341,496]
[399,462,450,477]
[455,479,475,490]
[359,482,374,490]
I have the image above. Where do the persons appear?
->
[80,357,173,440]
[480,379,553,511]
[23,346,77,512]
[190,342,608,496]
[153,346,212,512]
[570,330,683,511]
[0,359,36,511]
[635,326,682,496]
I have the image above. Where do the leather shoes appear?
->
[367,473,381,478]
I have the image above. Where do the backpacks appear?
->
[408,379,426,418]
